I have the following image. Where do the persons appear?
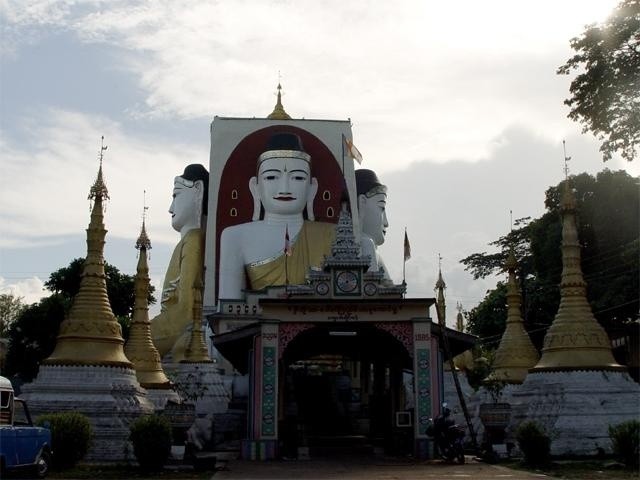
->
[147,163,207,357]
[354,168,397,284]
[217,134,338,300]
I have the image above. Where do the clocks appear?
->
[336,271,358,293]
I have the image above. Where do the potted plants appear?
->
[473,335,512,432]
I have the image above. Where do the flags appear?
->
[404,231,411,262]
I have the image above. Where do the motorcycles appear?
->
[425,401,466,464]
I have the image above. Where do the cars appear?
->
[0,373,53,478]
